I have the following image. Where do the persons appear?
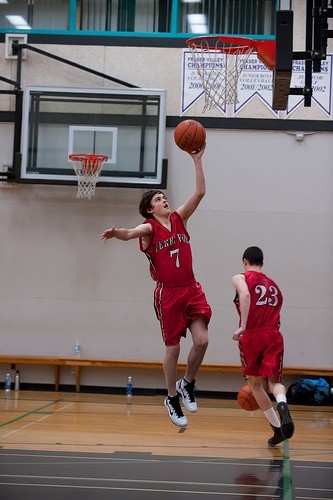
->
[231,246,295,446]
[101,142,212,427]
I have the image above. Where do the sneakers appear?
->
[277,402,294,439]
[164,394,188,426]
[268,423,283,446]
[175,376,198,412]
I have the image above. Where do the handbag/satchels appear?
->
[285,377,331,406]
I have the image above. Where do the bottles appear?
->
[13,369,20,390]
[5,372,12,391]
[74,341,81,357]
[127,376,133,398]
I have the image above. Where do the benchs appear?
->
[0,356,333,415]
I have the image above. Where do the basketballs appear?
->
[173,120,207,152]
[237,385,260,411]
